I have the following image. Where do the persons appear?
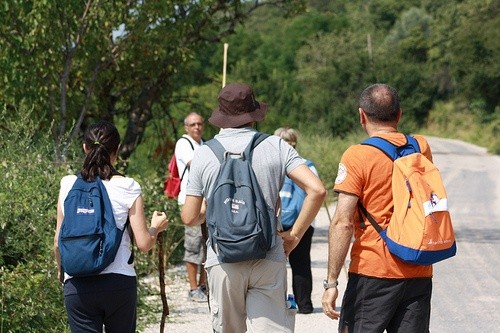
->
[272,126,322,315]
[51,120,171,332]
[324,84,435,333]
[173,111,211,304]
[181,84,328,332]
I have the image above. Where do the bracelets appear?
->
[323,280,340,291]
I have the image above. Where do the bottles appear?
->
[286,294,297,309]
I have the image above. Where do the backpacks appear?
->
[164,137,206,199]
[357,133,457,264]
[58,173,134,283]
[206,131,273,263]
[279,159,313,228]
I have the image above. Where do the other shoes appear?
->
[287,294,300,313]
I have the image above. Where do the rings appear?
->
[326,309,330,313]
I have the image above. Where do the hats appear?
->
[208,84,266,128]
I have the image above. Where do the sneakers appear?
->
[188,285,209,302]
